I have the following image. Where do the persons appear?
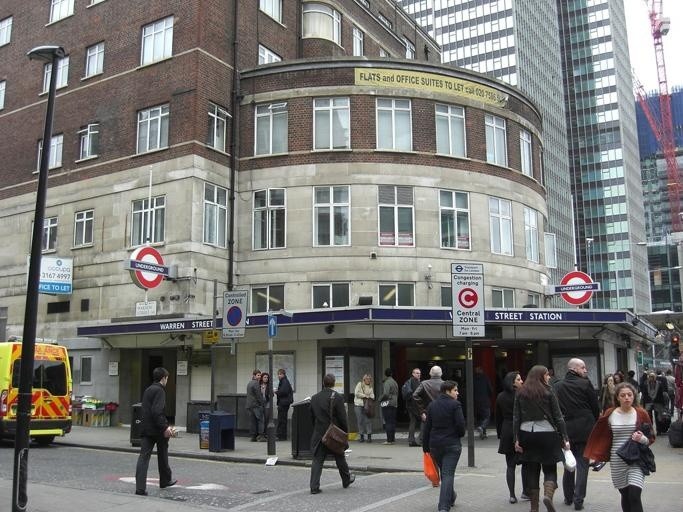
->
[496,358,600,512]
[355,373,375,442]
[245,369,294,442]
[403,366,444,447]
[378,368,399,445]
[136,367,177,495]
[472,365,493,440]
[307,374,355,494]
[584,383,656,512]
[423,381,465,512]
[600,369,676,435]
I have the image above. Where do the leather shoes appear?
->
[311,488,321,493]
[343,476,354,488]
[136,489,147,495]
[161,479,176,488]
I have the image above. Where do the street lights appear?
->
[585,237,596,308]
[11,44,66,512]
[613,241,648,309]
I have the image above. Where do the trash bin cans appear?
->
[130,402,145,447]
[208,410,236,452]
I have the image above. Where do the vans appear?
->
[0,335,72,446]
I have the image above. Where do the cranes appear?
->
[632,0,683,234]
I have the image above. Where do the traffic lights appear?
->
[670,333,680,362]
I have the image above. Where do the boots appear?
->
[543,481,556,512]
[529,488,539,512]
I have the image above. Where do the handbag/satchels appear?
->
[363,398,375,418]
[565,414,596,443]
[321,424,348,455]
[402,378,413,401]
[423,453,440,484]
